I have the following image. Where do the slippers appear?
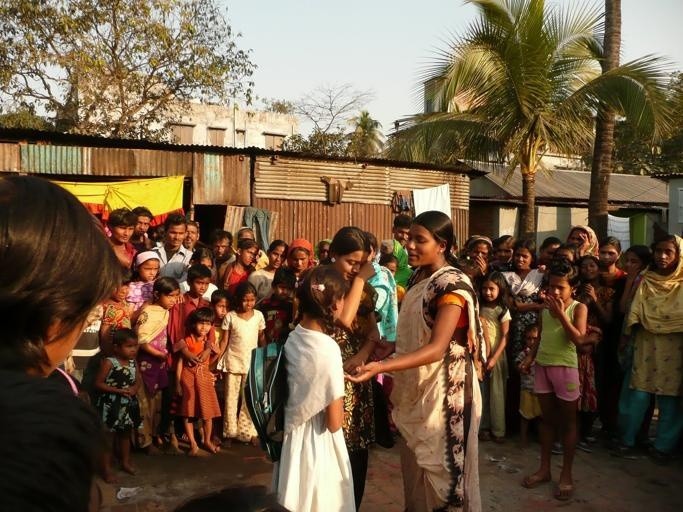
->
[144,426,222,460]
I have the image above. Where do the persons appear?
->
[0,175,122,512]
[60,207,683,511]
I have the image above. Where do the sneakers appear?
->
[475,427,678,503]
